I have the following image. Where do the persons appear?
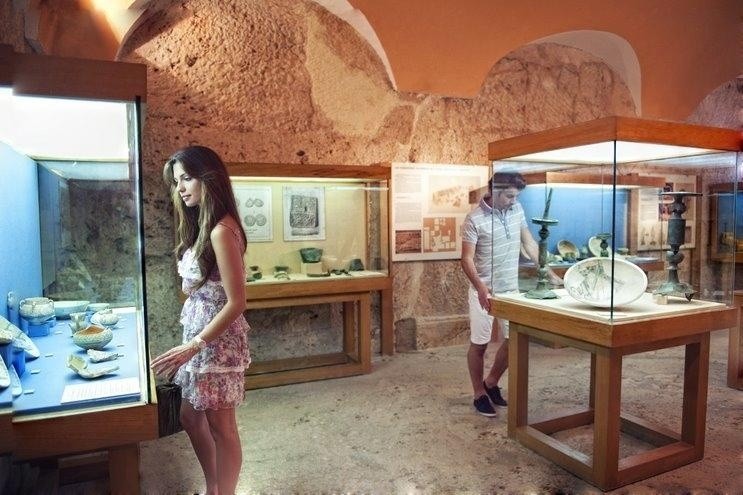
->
[460,172,563,418]
[151,146,248,495]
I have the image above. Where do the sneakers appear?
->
[483,380,508,408]
[474,395,497,417]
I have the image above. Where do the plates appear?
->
[556,239,580,262]
[563,257,649,310]
[586,236,610,257]
[0,352,11,390]
[8,364,21,396]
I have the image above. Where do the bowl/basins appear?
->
[299,248,322,263]
[0,314,39,359]
[72,327,113,350]
[53,299,89,320]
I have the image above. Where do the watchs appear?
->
[192,336,206,351]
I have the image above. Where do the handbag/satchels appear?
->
[156,383,184,438]
